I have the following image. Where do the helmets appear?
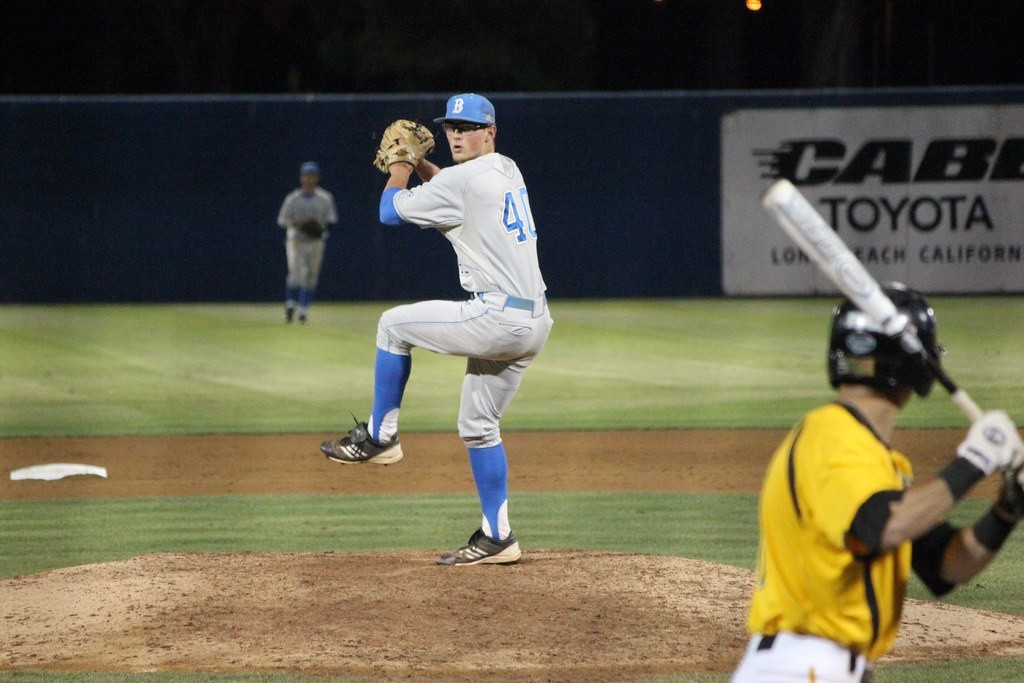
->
[828,283,942,404]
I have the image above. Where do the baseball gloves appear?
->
[371,118,436,174]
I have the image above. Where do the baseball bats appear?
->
[759,177,985,425]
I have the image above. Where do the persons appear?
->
[319,92,551,565]
[277,162,337,325]
[727,280,1024,683]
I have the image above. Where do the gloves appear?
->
[956,408,1024,477]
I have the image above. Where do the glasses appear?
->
[441,123,491,132]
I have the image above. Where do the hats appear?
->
[300,161,319,173]
[434,93,495,126]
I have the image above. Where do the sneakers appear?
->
[320,413,403,466]
[285,305,306,321]
[436,527,522,566]
